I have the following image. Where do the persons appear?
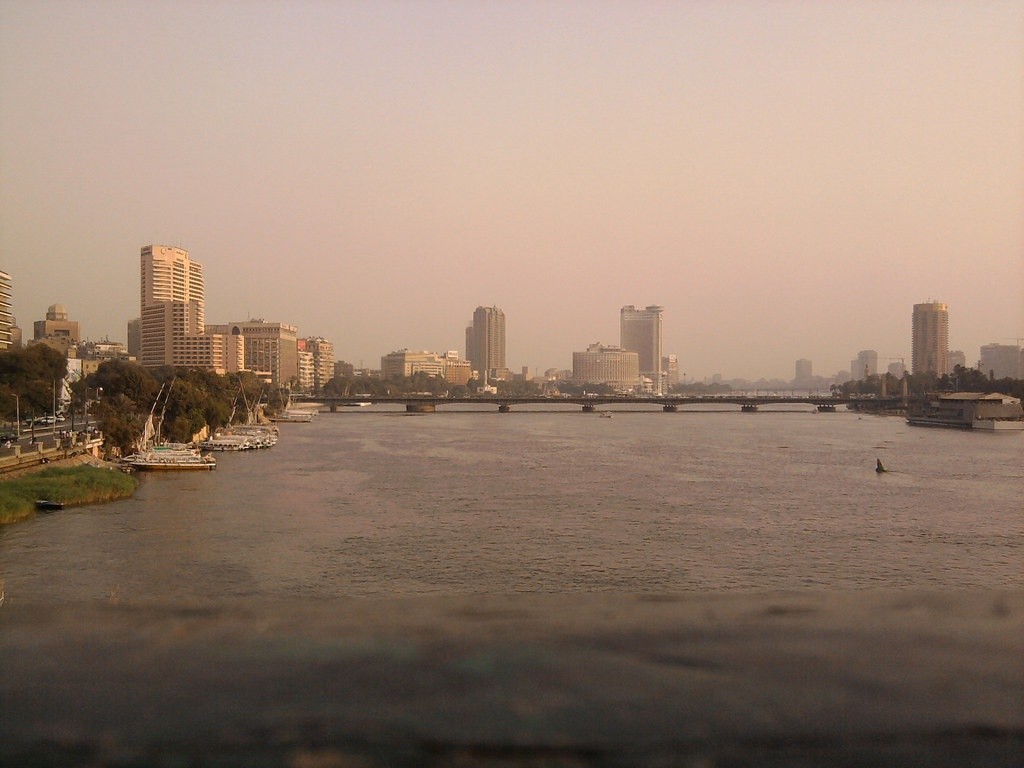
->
[76,425,97,436]
[29,437,37,446]
[5,440,11,451]
[60,430,72,439]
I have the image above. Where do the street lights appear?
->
[85,388,92,428]
[47,387,56,433]
[96,387,103,399]
[11,392,20,442]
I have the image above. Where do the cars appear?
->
[42,415,56,425]
[61,398,71,405]
[56,414,65,423]
[0,434,19,443]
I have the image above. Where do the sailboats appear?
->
[118,373,281,470]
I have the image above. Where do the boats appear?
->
[287,409,318,416]
[598,410,614,419]
[269,414,311,423]
[35,500,65,513]
